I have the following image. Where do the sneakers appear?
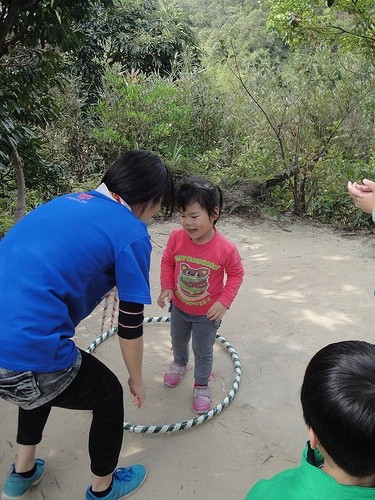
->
[163,361,189,387]
[2,457,47,499]
[192,383,212,414]
[85,463,147,499]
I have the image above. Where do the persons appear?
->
[0,151,176,500]
[244,340,375,500]
[348,179,375,223]
[157,180,244,414]
